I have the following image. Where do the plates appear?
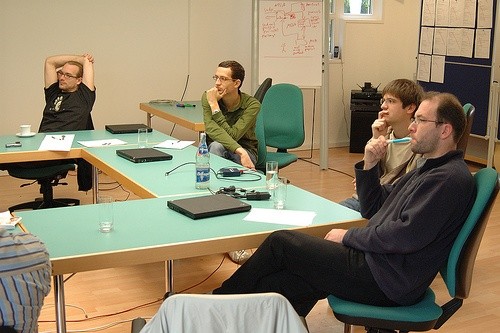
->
[16,132,36,137]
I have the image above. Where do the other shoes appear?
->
[228,249,252,264]
[163,291,176,301]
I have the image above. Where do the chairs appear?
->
[267,83,305,169]
[253,78,272,103]
[328,167,500,333]
[255,106,266,167]
[458,103,475,159]
[9,165,81,212]
[155,292,288,333]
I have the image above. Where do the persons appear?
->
[162,92,477,319]
[0,227,51,333]
[201,61,262,170]
[0,53,96,171]
[228,79,425,264]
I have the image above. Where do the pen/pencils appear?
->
[172,140,181,144]
[52,136,62,140]
[370,136,412,143]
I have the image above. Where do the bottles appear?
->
[334,46,339,58]
[195,134,210,190]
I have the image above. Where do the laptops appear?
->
[167,194,251,220]
[116,148,172,163]
[105,124,152,134]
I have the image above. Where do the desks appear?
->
[0,101,369,333]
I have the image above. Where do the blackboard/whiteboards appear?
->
[252,0,329,90]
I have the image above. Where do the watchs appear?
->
[212,109,221,115]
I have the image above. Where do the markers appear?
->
[176,104,196,108]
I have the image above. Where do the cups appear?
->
[20,125,31,135]
[138,128,148,142]
[273,177,287,211]
[98,195,114,232]
[266,161,278,191]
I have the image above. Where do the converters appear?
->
[220,167,241,177]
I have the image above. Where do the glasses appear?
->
[57,70,79,79]
[410,117,447,124]
[213,75,237,82]
[380,98,403,104]
